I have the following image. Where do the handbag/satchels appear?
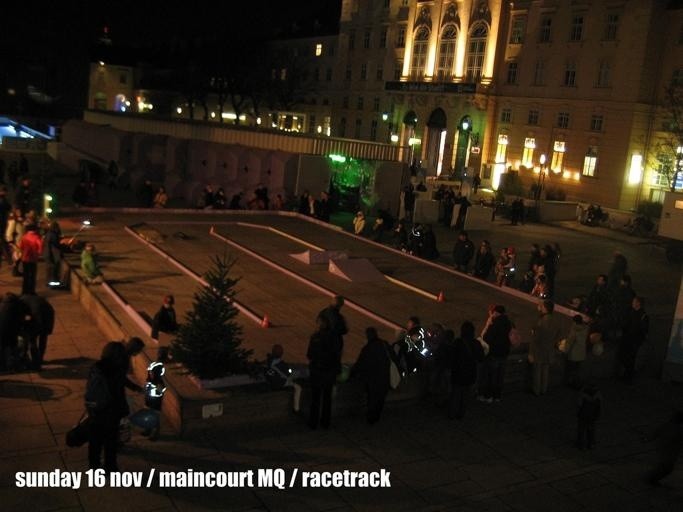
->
[64,407,89,449]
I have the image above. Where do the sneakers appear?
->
[479,396,502,404]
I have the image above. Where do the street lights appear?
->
[533,151,546,208]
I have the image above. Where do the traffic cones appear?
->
[438,289,446,303]
[262,312,270,328]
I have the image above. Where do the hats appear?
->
[164,295,175,305]
